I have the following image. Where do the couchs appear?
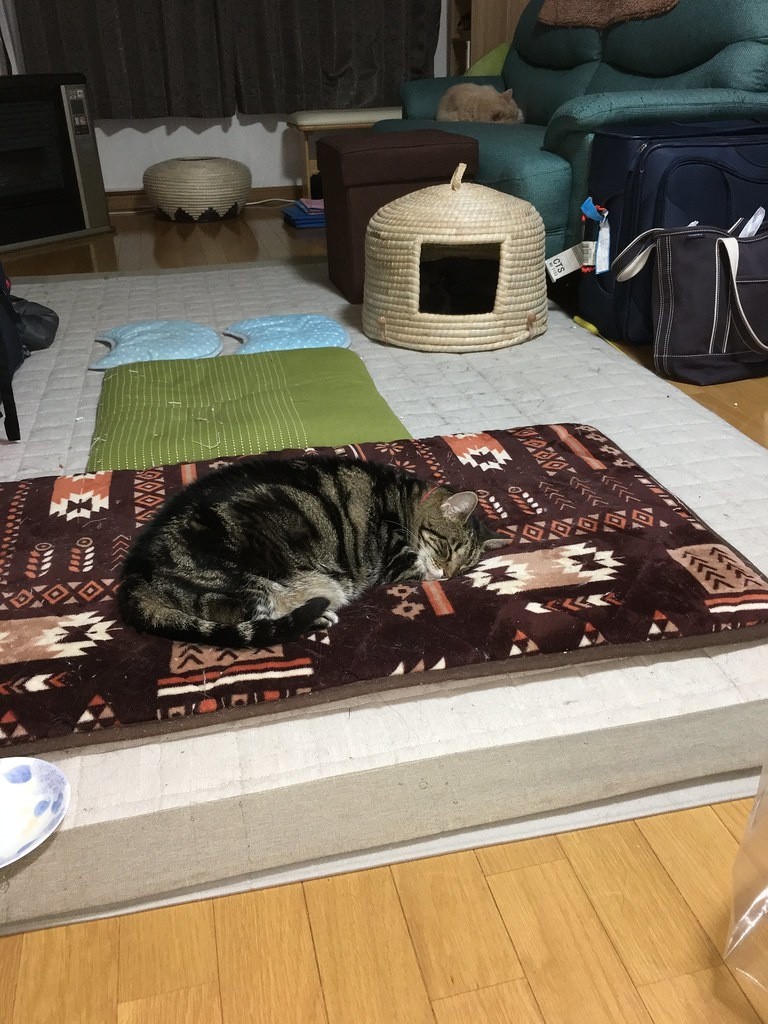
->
[373,0,767,259]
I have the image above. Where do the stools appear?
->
[287,106,403,199]
[317,129,480,304]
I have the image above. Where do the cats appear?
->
[117,452,516,649]
[435,82,526,125]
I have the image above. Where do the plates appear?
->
[1,756,72,868]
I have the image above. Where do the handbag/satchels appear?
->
[611,222,768,386]
[542,113,768,344]
